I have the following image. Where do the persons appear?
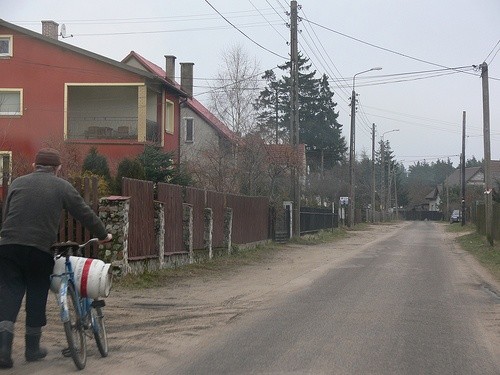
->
[0,147,112,369]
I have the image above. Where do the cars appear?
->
[450,210,462,224]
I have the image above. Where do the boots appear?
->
[25,333,48,361]
[0,330,14,368]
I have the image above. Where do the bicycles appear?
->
[50,238,113,369]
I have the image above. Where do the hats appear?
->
[35,147,61,166]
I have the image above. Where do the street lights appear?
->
[350,66,382,228]
[382,129,400,221]
